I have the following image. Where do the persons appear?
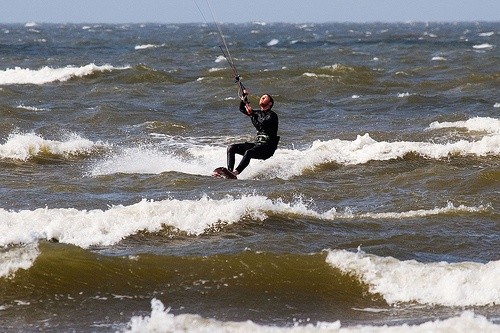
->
[213,89,279,179]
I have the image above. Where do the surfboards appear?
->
[213,166,237,179]
